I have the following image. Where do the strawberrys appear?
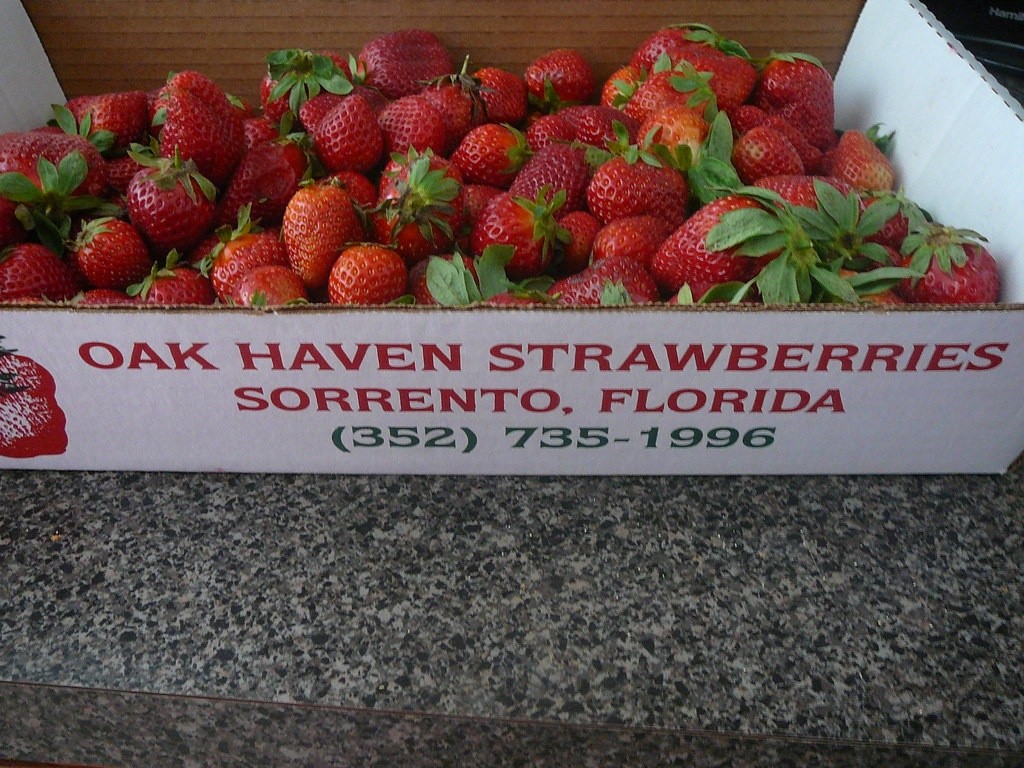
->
[0,23,1000,308]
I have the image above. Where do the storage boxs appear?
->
[0,2,1023,475]
